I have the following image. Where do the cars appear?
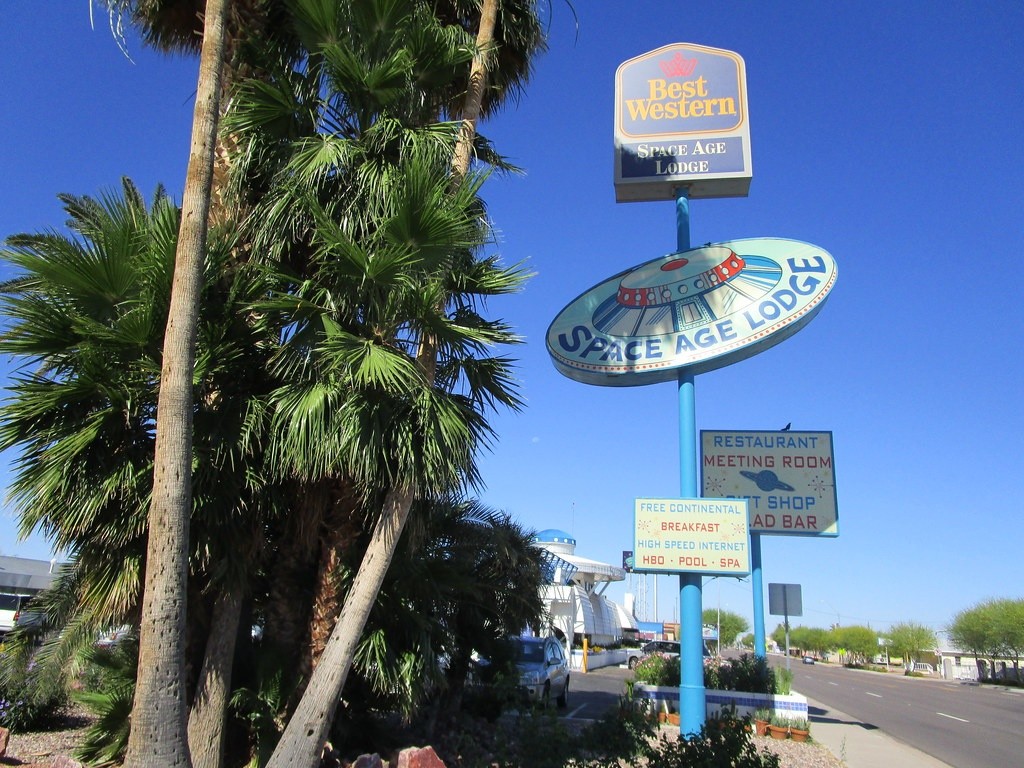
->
[803,656,814,665]
[473,638,570,717]
[626,640,712,671]
